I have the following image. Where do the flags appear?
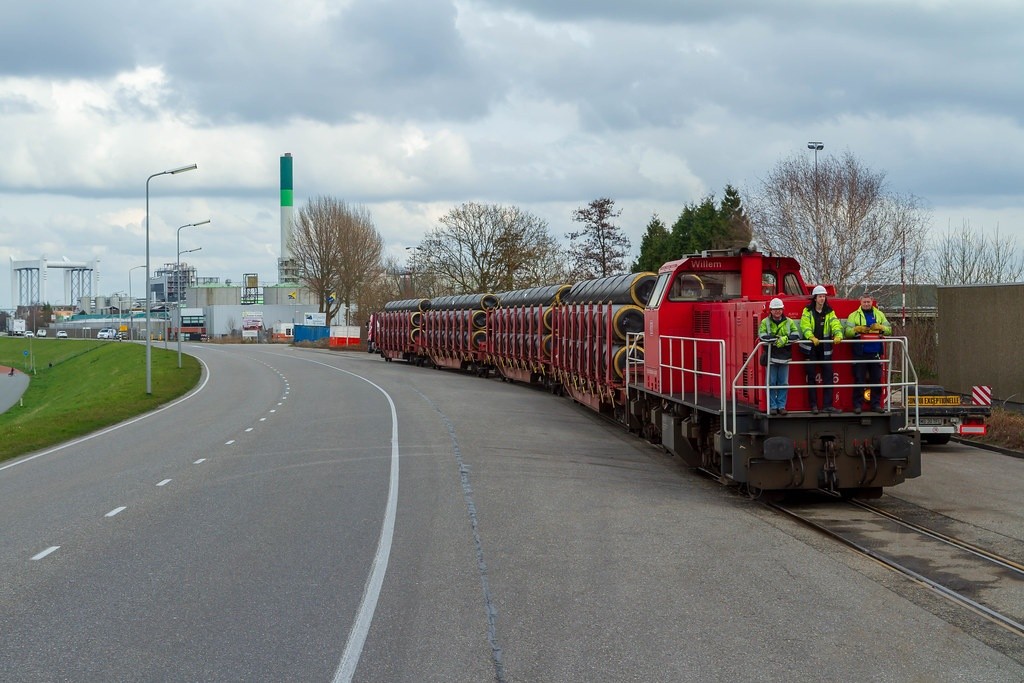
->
[288,292,297,299]
[327,292,338,302]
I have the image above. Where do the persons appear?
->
[758,298,802,415]
[846,291,893,414]
[800,285,845,414]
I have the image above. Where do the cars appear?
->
[24,330,35,339]
[56,331,67,338]
[116,331,129,340]
[36,329,47,338]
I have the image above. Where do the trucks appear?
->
[7,318,24,336]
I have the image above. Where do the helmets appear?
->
[812,285,827,296]
[769,298,785,309]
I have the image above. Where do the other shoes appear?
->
[822,407,842,413]
[778,409,787,415]
[872,407,885,413]
[812,406,818,413]
[770,409,778,415]
[855,407,861,414]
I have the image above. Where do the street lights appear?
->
[129,265,146,340]
[808,141,824,285]
[146,164,197,394]
[406,246,423,299]
[177,217,211,368]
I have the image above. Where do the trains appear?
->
[368,246,924,501]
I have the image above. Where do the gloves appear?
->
[775,336,789,347]
[855,326,869,334]
[809,337,820,346]
[870,323,884,331]
[833,338,840,345]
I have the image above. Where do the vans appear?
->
[97,329,116,340]
